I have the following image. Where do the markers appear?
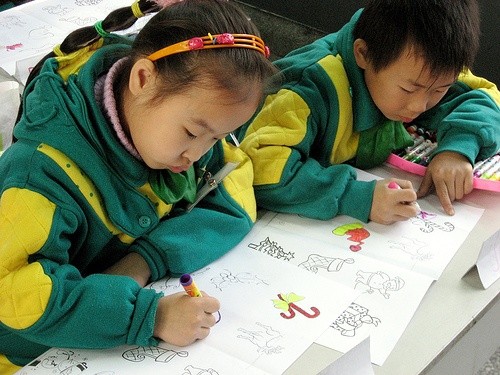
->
[387,181,425,215]
[391,125,500,182]
[179,275,203,298]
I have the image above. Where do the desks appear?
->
[15,165,500,375]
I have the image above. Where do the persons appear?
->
[0,0,271,375]
[226,0,499,225]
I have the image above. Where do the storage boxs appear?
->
[385,153,500,192]
